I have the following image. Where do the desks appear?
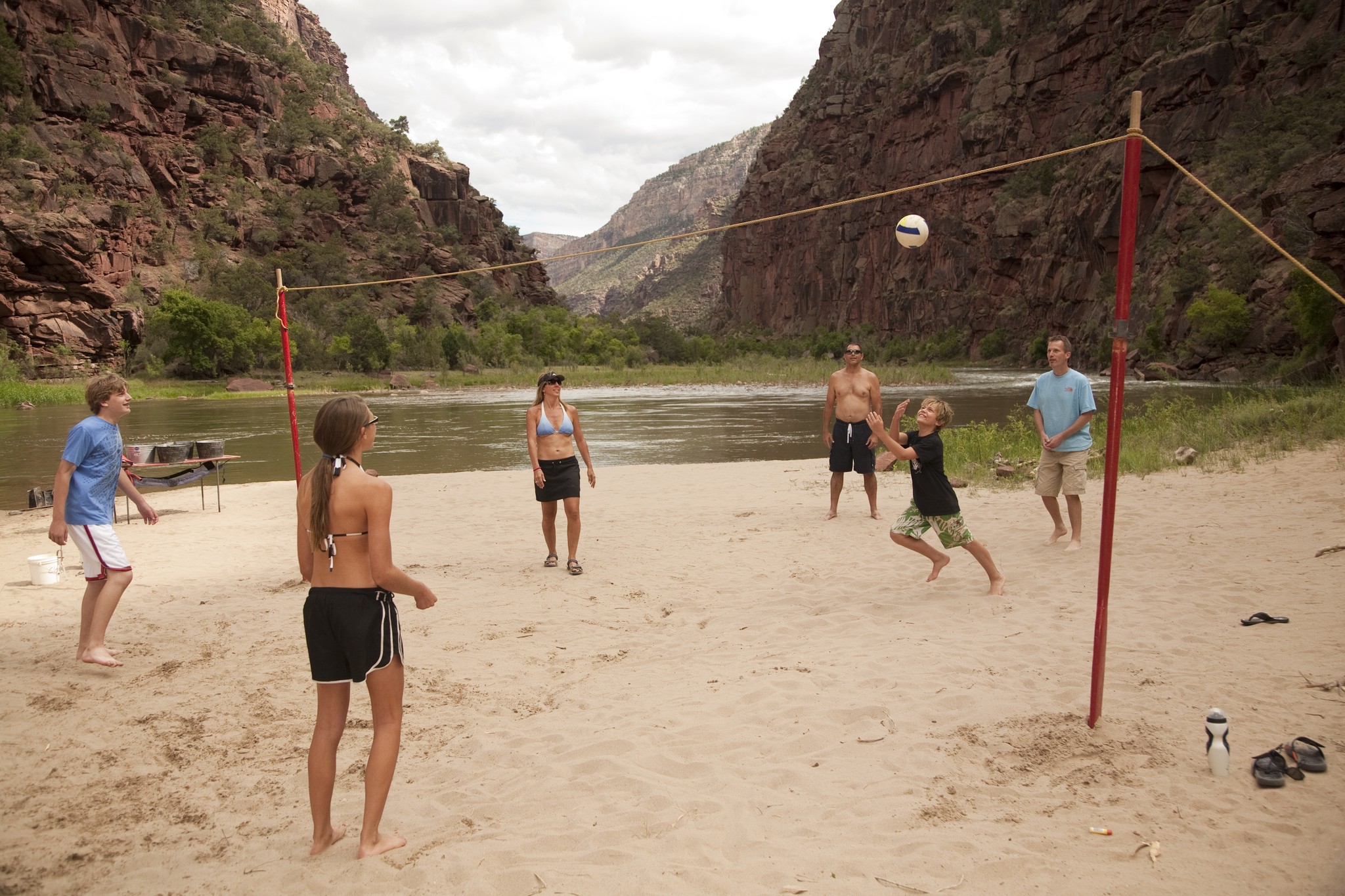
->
[114,455,241,525]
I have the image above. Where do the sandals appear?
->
[567,559,583,575]
[544,553,558,567]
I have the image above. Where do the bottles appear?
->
[1205,707,1230,775]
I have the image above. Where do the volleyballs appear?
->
[895,214,929,249]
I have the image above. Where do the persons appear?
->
[48,372,160,667]
[526,371,596,575]
[1026,336,1097,552]
[865,397,1005,596]
[296,395,439,860]
[823,341,885,521]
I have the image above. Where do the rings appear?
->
[535,481,538,483]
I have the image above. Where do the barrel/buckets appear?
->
[28,554,62,586]
[155,442,189,464]
[164,441,194,458]
[195,439,225,458]
[124,444,155,464]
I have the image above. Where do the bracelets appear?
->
[533,466,541,471]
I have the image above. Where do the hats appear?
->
[538,372,565,387]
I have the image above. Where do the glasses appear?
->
[845,350,862,355]
[363,415,379,427]
[547,379,562,385]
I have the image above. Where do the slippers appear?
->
[1241,612,1289,625]
[1251,749,1286,787]
[1284,736,1327,772]
